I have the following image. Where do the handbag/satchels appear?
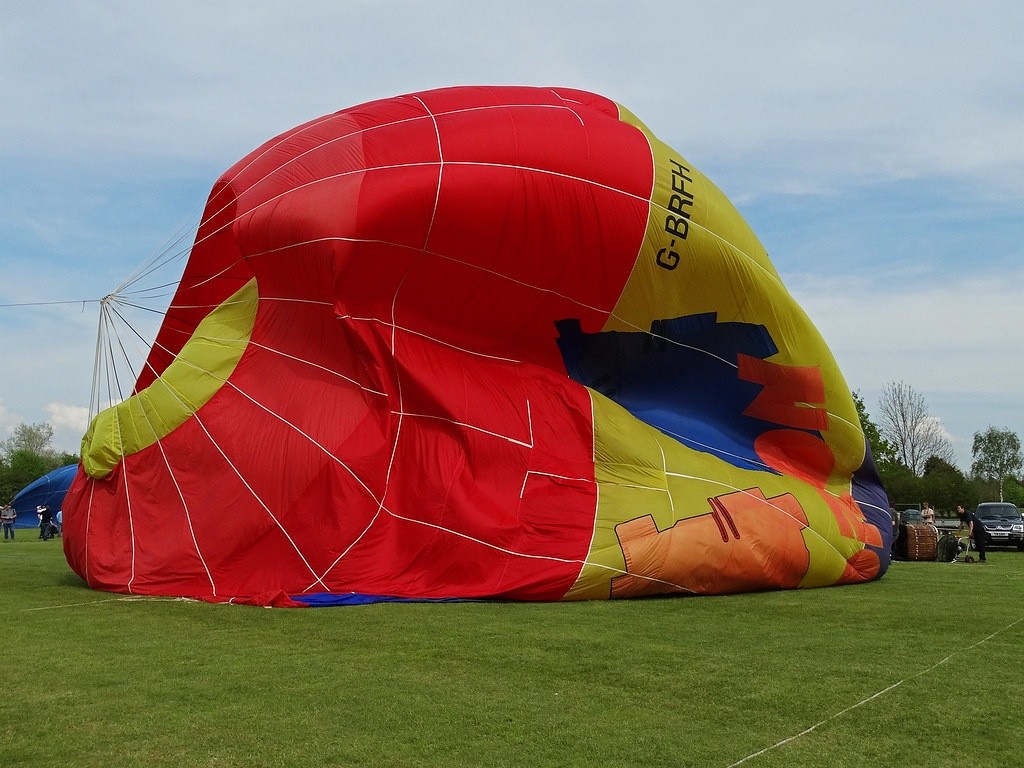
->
[13,509,15,524]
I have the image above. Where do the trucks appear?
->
[900,509,964,535]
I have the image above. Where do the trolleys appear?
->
[936,535,975,563]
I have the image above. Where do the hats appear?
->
[7,504,12,506]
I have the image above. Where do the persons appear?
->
[956,505,991,563]
[921,502,934,524]
[889,501,900,565]
[35,504,63,542]
[0,504,17,541]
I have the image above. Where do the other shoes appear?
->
[977,559,985,562]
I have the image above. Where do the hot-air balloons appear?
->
[58,85,894,607]
[5,462,79,529]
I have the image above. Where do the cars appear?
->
[969,502,1024,551]
